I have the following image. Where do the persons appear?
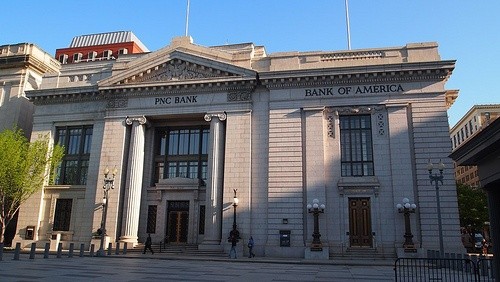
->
[247,236,255,258]
[460,224,494,253]
[143,234,155,254]
[228,235,238,259]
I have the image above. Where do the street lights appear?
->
[94,165,118,257]
[228,189,244,243]
[427,162,447,261]
[96,197,110,236]
[306,199,327,250]
[397,197,419,253]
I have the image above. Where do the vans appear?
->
[463,233,485,249]
